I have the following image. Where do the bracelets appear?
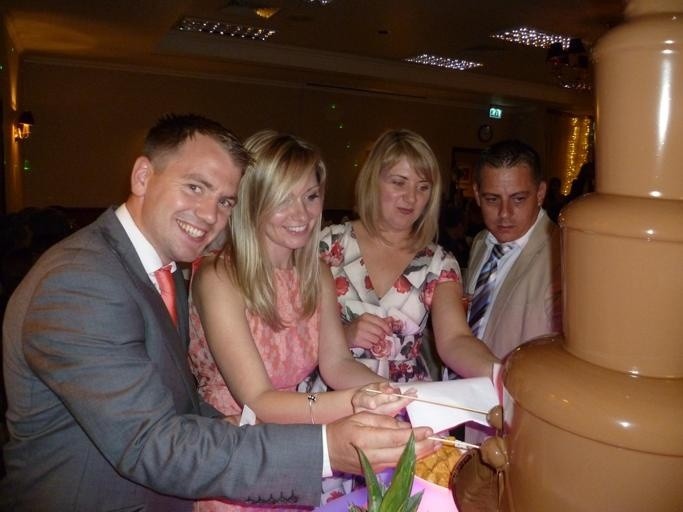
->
[304,392,318,427]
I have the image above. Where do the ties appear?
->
[155,265,178,326]
[448,243,512,380]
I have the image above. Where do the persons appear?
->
[439,135,563,443]
[0,110,445,511]
[296,126,506,505]
[187,129,420,512]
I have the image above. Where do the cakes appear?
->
[449,0,683,512]
